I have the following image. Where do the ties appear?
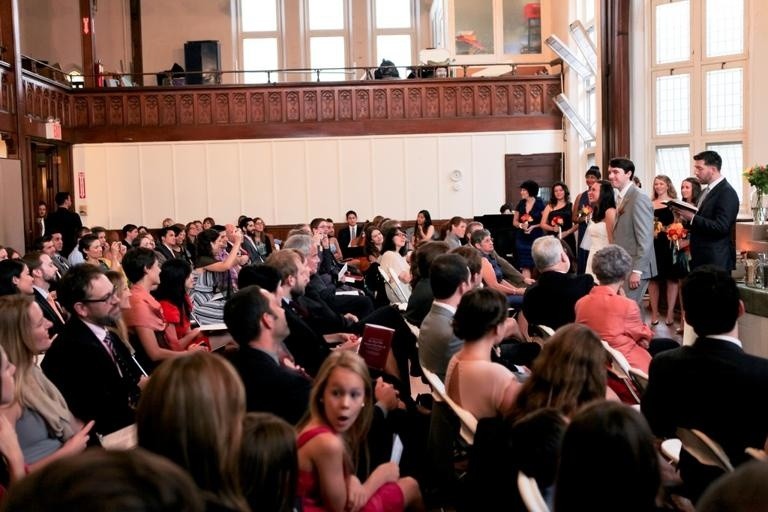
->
[351,227,356,242]
[47,294,59,316]
[104,332,135,382]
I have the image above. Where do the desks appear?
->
[736,222,768,251]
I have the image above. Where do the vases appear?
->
[749,185,768,225]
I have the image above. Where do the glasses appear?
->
[479,238,494,243]
[81,282,117,302]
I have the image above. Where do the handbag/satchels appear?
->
[100,421,141,454]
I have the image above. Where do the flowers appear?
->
[576,205,593,223]
[665,222,692,272]
[551,216,564,232]
[742,163,768,220]
[520,214,534,230]
[739,251,760,266]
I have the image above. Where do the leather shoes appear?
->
[650,312,684,334]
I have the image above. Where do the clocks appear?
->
[449,168,463,191]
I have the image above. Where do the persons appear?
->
[3,150,768,512]
